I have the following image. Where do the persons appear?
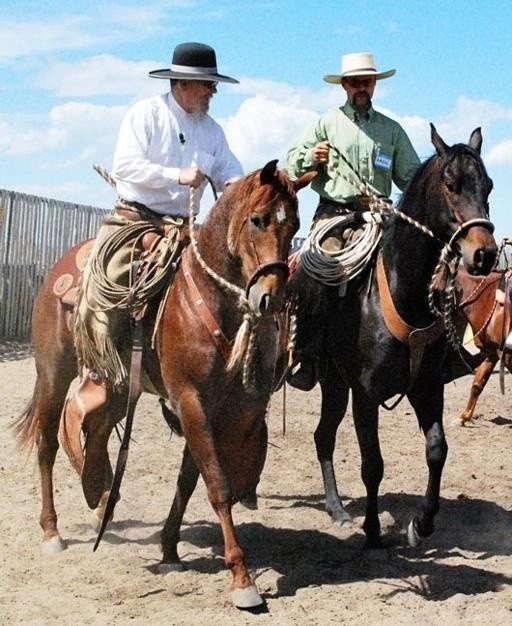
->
[69,41,245,383]
[282,51,424,388]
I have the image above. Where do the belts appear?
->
[116,197,189,224]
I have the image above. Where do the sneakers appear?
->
[288,363,327,390]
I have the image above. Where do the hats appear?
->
[149,42,240,83]
[323,53,395,84]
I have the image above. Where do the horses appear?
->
[433,258,512,428]
[1,158,319,609]
[280,122,499,564]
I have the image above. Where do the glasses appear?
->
[345,78,373,88]
[182,80,219,89]
